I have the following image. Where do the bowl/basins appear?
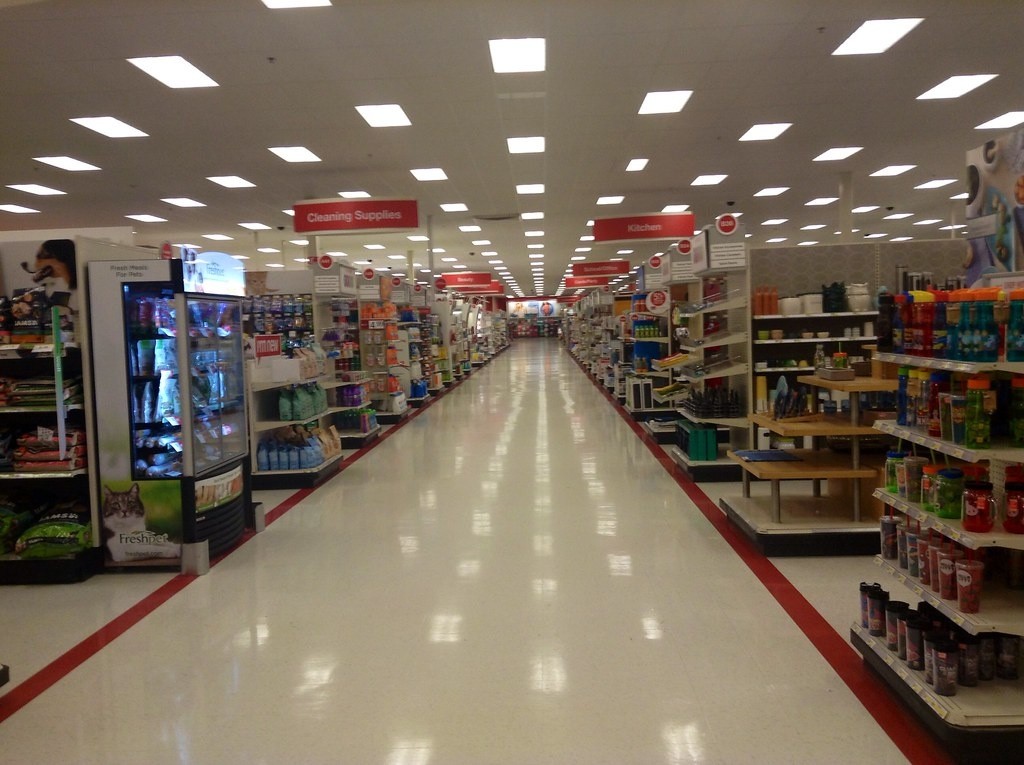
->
[758,331,769,340]
[799,360,808,367]
[755,362,767,368]
[817,332,829,338]
[802,333,813,338]
[771,330,784,339]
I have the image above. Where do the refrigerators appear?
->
[88,251,263,575]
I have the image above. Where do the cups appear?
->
[844,328,851,337]
[864,320,874,337]
[852,328,860,337]
[879,515,984,614]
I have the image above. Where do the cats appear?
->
[101,482,181,564]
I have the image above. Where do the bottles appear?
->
[242,295,313,332]
[755,286,778,316]
[342,384,369,406]
[897,368,940,437]
[635,357,647,373]
[337,345,360,371]
[878,287,1024,362]
[387,346,397,364]
[635,325,658,337]
[389,374,398,392]
[634,299,649,312]
[385,323,398,340]
[334,409,377,432]
[813,344,825,378]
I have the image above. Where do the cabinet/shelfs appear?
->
[0,236,1024,765]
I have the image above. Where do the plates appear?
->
[775,375,788,418]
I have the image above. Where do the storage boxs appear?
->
[817,367,854,382]
